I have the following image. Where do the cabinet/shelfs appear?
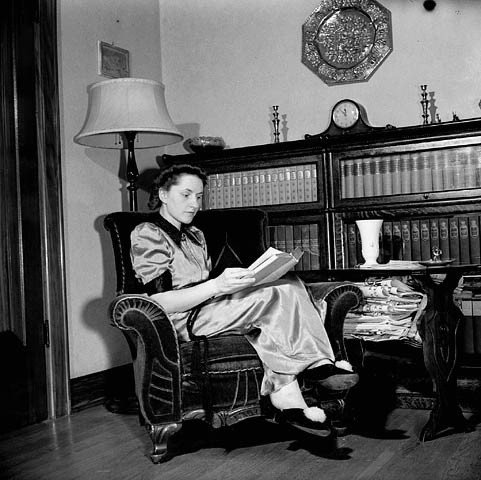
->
[323,135,481,384]
[198,158,331,278]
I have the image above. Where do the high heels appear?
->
[297,361,360,392]
[264,394,331,436]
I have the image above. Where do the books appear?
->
[201,163,319,210]
[341,210,480,268]
[263,222,320,271]
[458,288,481,353]
[338,144,481,200]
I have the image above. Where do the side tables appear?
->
[291,260,481,441]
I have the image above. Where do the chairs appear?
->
[100,207,364,462]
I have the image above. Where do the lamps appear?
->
[74,77,185,212]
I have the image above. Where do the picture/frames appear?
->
[97,40,130,79]
[300,0,394,86]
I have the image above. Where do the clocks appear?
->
[331,101,360,129]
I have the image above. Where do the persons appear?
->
[129,162,361,437]
[239,246,304,287]
